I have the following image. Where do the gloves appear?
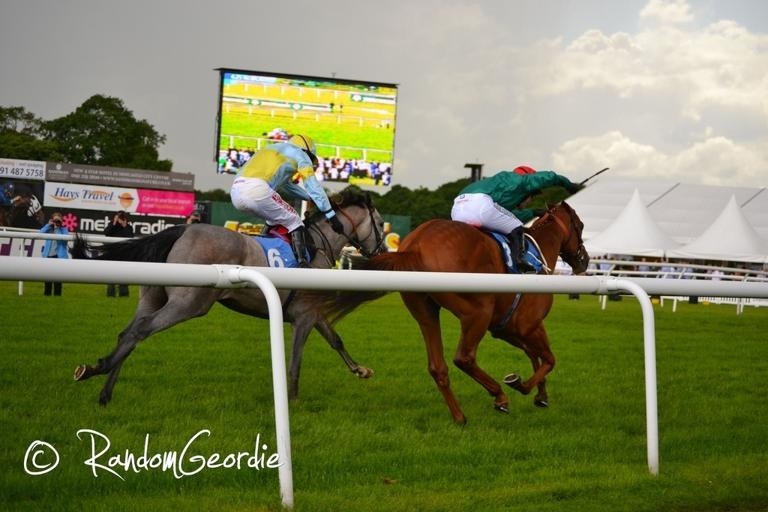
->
[328,216,344,235]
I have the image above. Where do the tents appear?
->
[663,193,767,265]
[583,189,680,259]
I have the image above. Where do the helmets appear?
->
[4,181,15,192]
[287,134,316,155]
[515,166,536,174]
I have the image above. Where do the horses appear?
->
[73,190,389,406]
[0,196,45,229]
[299,199,590,425]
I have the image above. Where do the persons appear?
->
[39,212,69,297]
[0,184,22,207]
[102,210,135,297]
[593,256,767,285]
[450,167,584,272]
[218,149,252,172]
[186,212,202,226]
[230,133,343,267]
[317,156,394,184]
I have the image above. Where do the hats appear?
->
[191,209,200,216]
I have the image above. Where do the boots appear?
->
[292,227,309,268]
[507,225,537,272]
